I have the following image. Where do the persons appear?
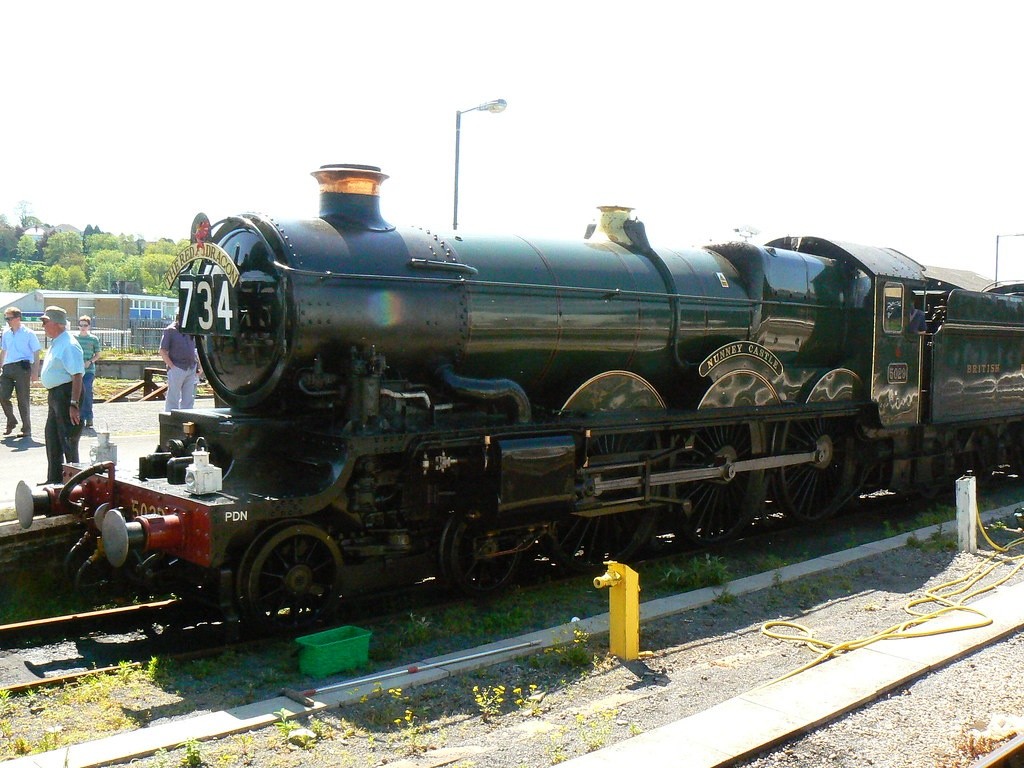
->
[158,308,203,412]
[0,306,42,436]
[37,306,85,487]
[72,315,101,429]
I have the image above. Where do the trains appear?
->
[16,161,1024,646]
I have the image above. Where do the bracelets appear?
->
[69,400,80,409]
[89,360,92,364]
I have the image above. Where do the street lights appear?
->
[452,98,508,230]
[994,233,1024,287]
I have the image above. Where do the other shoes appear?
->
[37,480,64,486]
[86,419,93,427]
[3,420,18,435]
[17,431,31,437]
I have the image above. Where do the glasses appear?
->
[78,324,89,328]
[4,316,15,321]
[42,319,50,325]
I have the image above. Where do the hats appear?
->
[39,306,68,324]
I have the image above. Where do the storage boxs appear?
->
[291,626,372,679]
